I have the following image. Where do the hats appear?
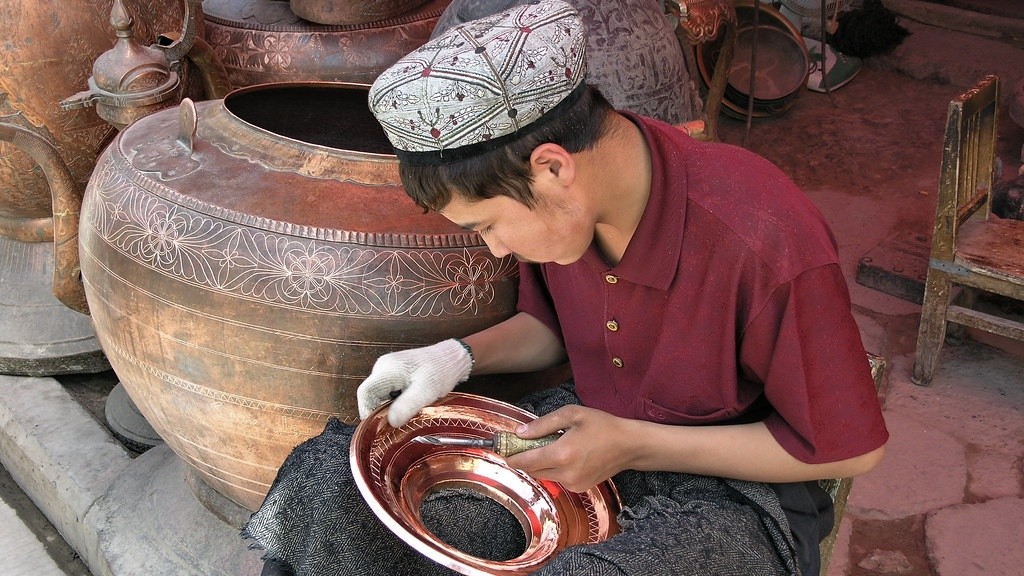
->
[367,1,591,164]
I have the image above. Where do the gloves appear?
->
[351,338,477,429]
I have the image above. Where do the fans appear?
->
[776,0,861,94]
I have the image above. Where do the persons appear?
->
[242,1,889,576]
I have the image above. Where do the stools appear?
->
[910,73,1024,387]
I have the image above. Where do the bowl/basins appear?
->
[696,0,810,122]
